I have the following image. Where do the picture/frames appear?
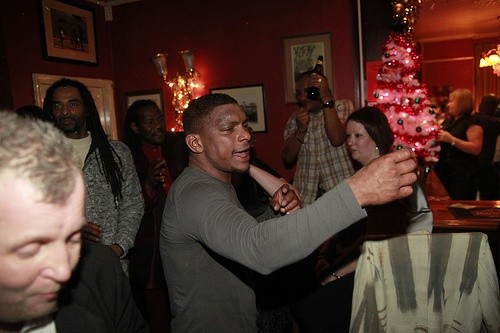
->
[209,84,268,135]
[125,89,163,114]
[32,72,117,136]
[40,0,99,68]
[283,32,335,104]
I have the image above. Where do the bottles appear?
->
[308,56,324,100]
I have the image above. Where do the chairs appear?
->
[349,232,500,333]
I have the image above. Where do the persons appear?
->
[0,80,500,333]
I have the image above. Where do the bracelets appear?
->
[332,272,340,279]
[293,133,303,144]
[322,96,330,102]
[451,137,456,145]
[297,129,307,133]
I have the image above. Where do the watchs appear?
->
[324,99,333,109]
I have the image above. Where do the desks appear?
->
[426,199,500,289]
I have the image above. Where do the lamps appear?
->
[389,0,422,42]
[479,44,500,80]
[150,50,205,132]
